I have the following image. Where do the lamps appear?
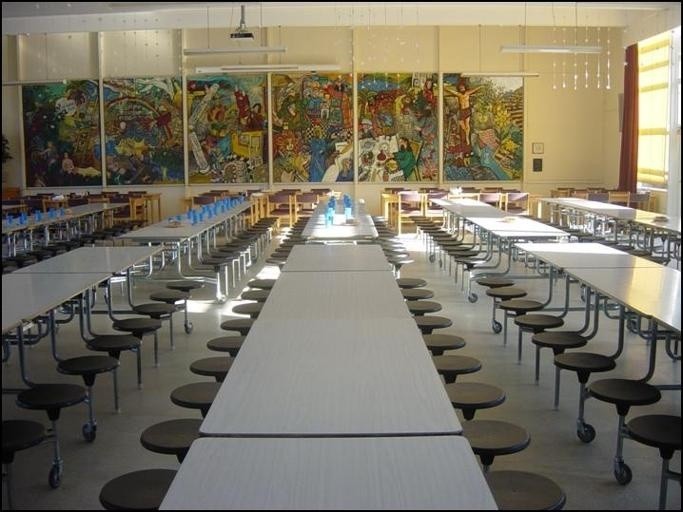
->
[2,34,66,91]
[497,1,606,58]
[183,0,288,56]
[459,22,541,80]
[193,1,342,73]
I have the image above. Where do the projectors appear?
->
[229,33,255,41]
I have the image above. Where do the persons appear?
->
[110,191,121,214]
[68,192,85,206]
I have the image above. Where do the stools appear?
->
[0,183,682,512]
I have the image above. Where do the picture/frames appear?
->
[532,143,544,154]
[531,157,542,173]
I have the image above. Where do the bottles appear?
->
[166,196,243,225]
[5,207,64,225]
[323,193,351,225]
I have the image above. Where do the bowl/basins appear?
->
[449,187,462,195]
